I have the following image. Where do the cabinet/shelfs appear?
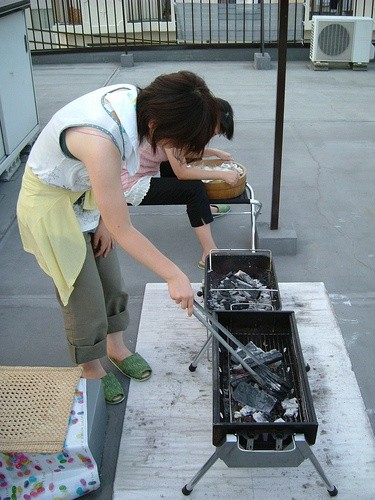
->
[0,0,42,182]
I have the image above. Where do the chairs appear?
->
[210,181,262,255]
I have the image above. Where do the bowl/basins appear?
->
[184,160,247,200]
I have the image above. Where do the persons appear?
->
[115,95,240,269]
[14,70,223,405]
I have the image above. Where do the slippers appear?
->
[210,204,231,218]
[107,352,152,382]
[197,260,205,270]
[98,371,125,404]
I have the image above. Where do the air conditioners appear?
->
[308,14,373,64]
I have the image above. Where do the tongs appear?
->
[189,300,292,398]
